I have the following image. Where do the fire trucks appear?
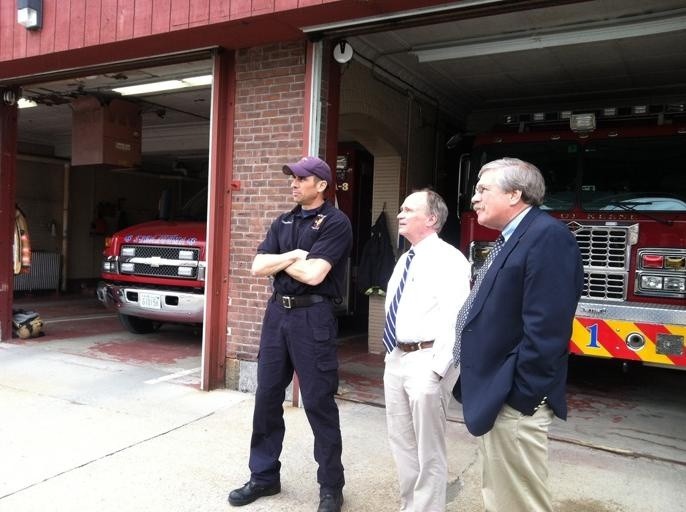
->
[96,141,375,336]
[445,101,686,373]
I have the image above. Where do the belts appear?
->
[397,341,433,352]
[275,293,323,309]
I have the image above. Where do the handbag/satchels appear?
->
[13,309,43,339]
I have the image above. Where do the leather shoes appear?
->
[229,481,280,506]
[318,493,343,512]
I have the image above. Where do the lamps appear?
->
[16,0,44,31]
[569,112,597,135]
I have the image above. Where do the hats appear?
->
[282,156,332,185]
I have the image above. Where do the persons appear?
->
[453,158,585,512]
[382,188,473,512]
[228,155,355,512]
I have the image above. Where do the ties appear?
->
[453,236,505,369]
[382,250,415,354]
